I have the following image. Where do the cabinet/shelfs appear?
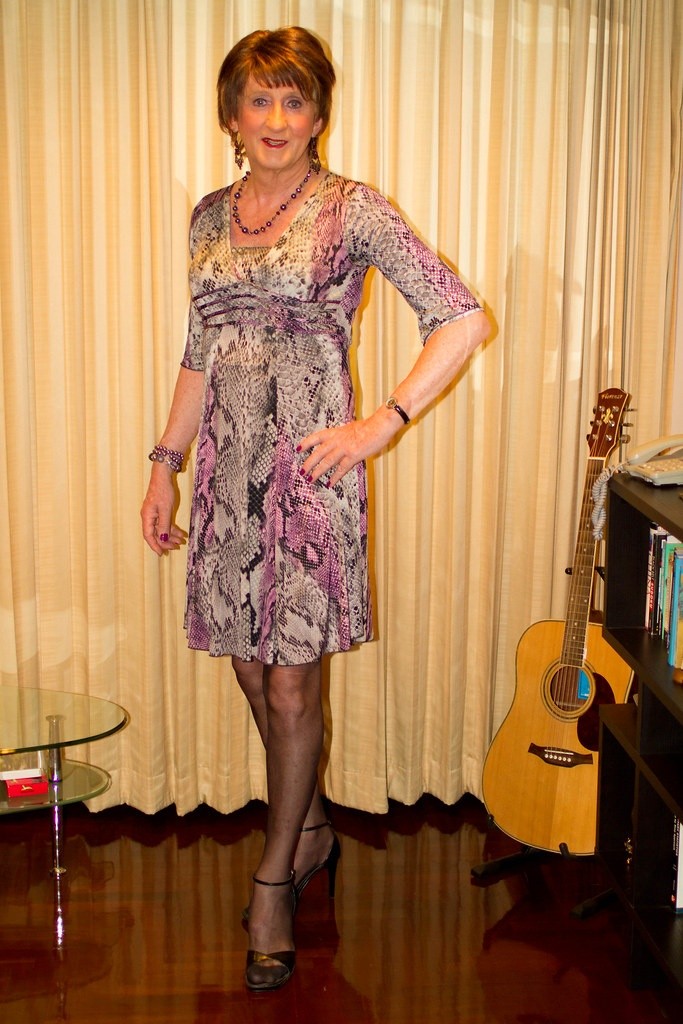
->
[592,473,683,1024]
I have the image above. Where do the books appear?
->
[640,521,683,668]
[667,816,683,914]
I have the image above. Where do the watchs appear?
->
[385,397,410,423]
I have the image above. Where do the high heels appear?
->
[241,874,300,994]
[241,821,343,926]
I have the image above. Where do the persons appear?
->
[141,26,485,989]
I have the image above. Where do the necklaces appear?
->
[232,168,312,234]
[149,445,184,474]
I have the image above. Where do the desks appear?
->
[0,684,131,949]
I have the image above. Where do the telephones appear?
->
[624,432,683,487]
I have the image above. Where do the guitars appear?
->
[477,385,641,863]
